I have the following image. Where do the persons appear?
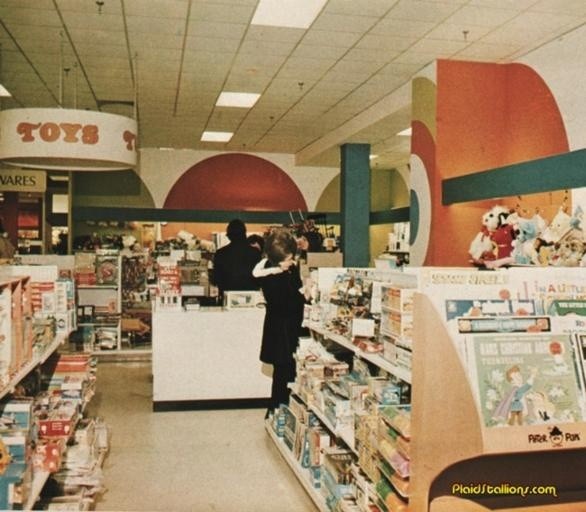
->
[244,235,266,252]
[297,228,325,269]
[251,231,312,421]
[212,217,262,306]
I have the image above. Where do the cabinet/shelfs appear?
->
[265,260,584,512]
[0,225,150,512]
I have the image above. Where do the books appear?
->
[418,266,585,455]
[268,394,381,512]
